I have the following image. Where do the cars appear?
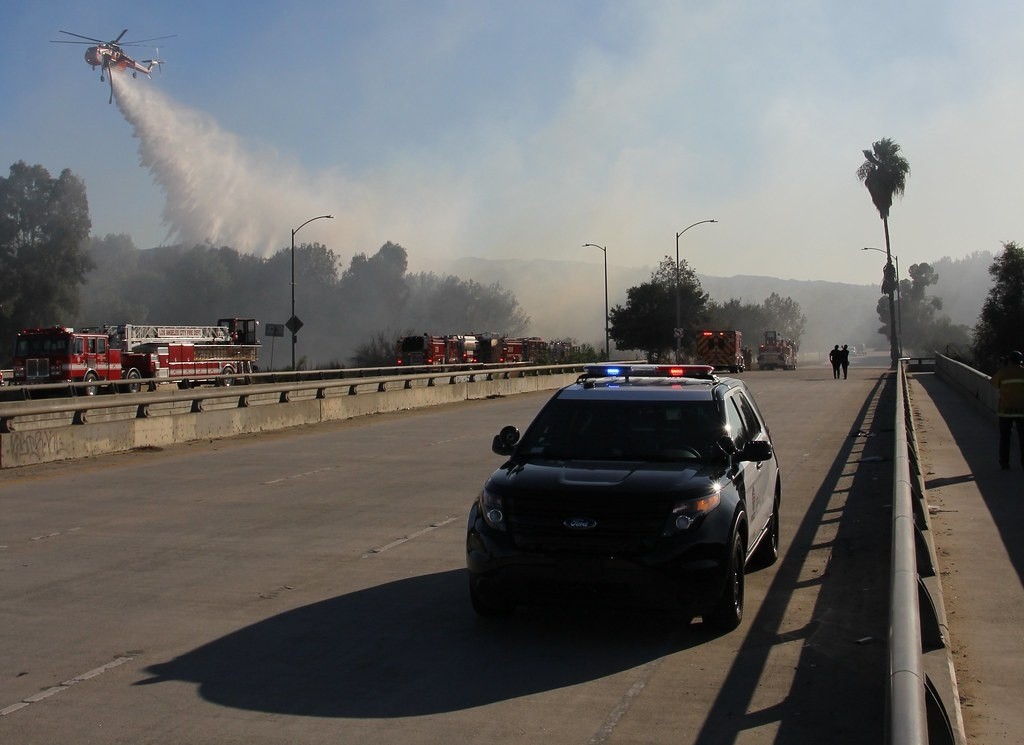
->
[847,346,856,356]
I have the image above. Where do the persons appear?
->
[990,350,1024,470]
[837,344,850,380]
[828,344,840,379]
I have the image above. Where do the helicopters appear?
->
[49,27,179,106]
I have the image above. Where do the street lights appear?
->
[580,241,610,362]
[862,246,903,361]
[674,218,719,365]
[290,214,333,372]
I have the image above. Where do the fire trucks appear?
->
[757,330,798,371]
[12,317,264,397]
[392,333,577,374]
[695,328,746,374]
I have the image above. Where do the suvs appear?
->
[466,359,782,638]
